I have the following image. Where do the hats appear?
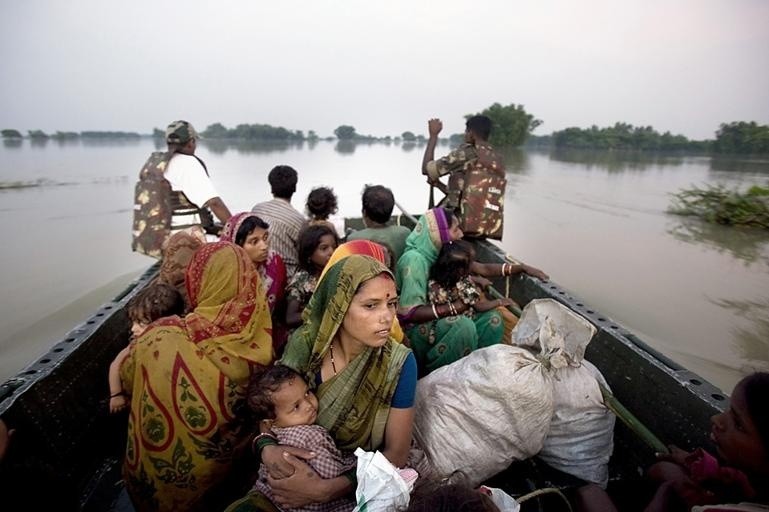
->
[165,119,200,146]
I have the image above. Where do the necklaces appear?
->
[328,342,342,375]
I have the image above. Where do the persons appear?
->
[301,239,410,347]
[130,121,233,262]
[241,365,467,512]
[222,211,294,362]
[403,485,500,512]
[578,372,769,511]
[346,184,412,263]
[109,241,274,511]
[421,115,507,240]
[305,187,337,233]
[286,225,337,326]
[159,231,203,308]
[392,206,550,370]
[223,255,417,512]
[109,283,184,412]
[251,165,307,276]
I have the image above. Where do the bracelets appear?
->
[111,392,124,398]
[251,432,277,454]
[509,265,512,274]
[451,303,458,315]
[501,263,507,276]
[447,300,453,317]
[431,303,440,320]
